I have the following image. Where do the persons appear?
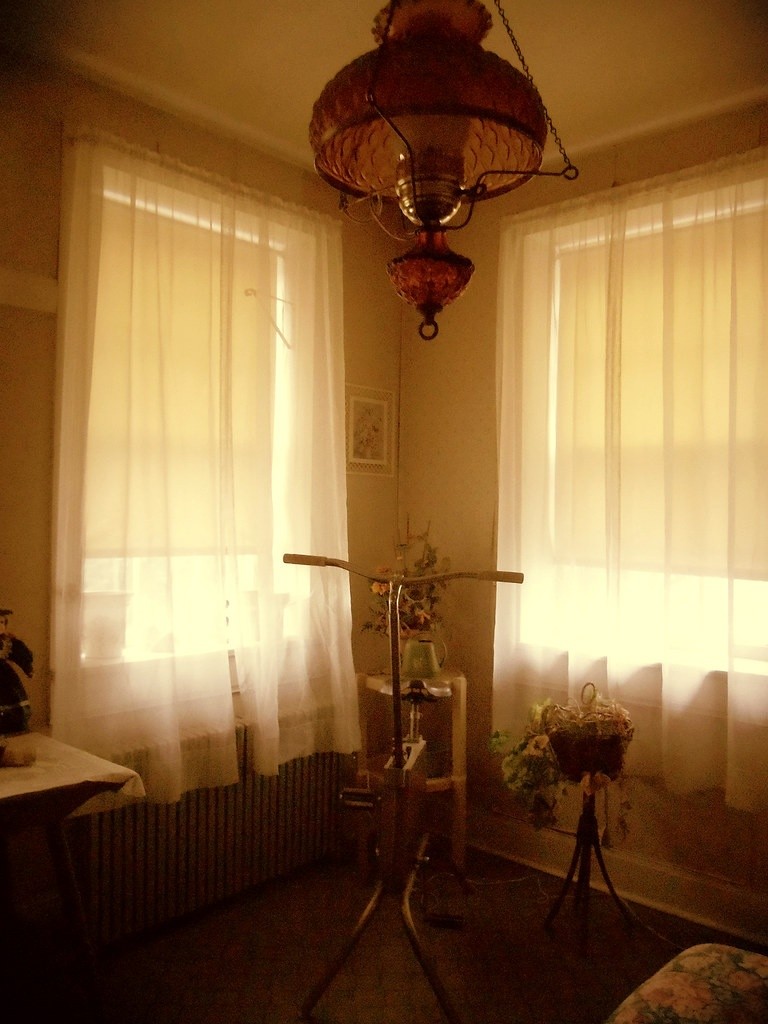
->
[0,609,35,735]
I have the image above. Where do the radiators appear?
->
[87,700,359,940]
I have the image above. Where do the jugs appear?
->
[403,632,448,678]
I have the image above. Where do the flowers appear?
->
[487,696,633,852]
[360,533,455,642]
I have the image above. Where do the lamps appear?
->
[308,0,579,341]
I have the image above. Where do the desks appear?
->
[0,733,146,971]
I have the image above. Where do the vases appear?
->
[546,719,633,783]
[398,633,448,679]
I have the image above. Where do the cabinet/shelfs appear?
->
[366,664,467,870]
